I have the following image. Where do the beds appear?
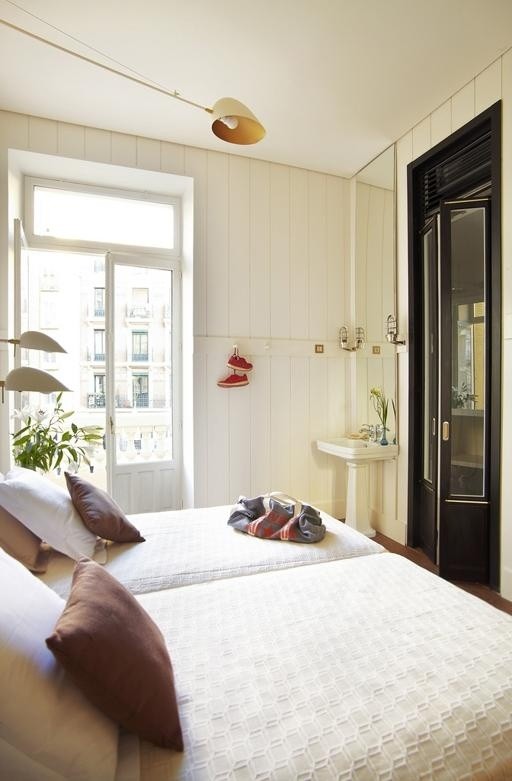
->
[0,489,512,779]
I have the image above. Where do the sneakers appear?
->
[226,352,253,372]
[216,373,250,388]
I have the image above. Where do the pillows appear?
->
[60,466,149,545]
[43,551,182,757]
[3,465,109,566]
[1,545,125,781]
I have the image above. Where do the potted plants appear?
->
[369,384,390,446]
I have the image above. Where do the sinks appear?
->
[317,439,399,459]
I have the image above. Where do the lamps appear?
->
[1,19,267,146]
[0,329,73,400]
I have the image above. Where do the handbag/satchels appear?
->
[226,487,326,543]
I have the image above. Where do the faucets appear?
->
[360,423,378,441]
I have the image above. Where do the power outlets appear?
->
[374,346,379,354]
[315,345,323,353]
